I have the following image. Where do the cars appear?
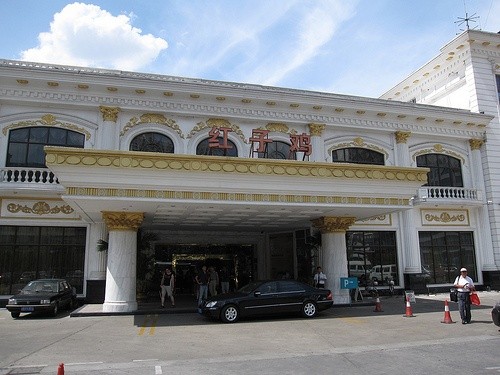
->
[491,300,500,328]
[6,279,77,318]
[204,279,334,323]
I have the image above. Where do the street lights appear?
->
[353,231,374,288]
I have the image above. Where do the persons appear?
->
[221,267,229,293]
[159,266,176,308]
[209,267,219,295]
[195,265,211,307]
[454,268,475,325]
[313,266,328,288]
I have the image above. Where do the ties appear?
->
[318,274,320,284]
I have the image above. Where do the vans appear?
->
[365,264,397,285]
[347,261,373,283]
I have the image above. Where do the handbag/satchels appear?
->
[450,287,457,302]
[469,290,480,306]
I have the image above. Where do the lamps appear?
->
[487,201,493,205]
[409,194,417,204]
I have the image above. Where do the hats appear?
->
[460,268,467,272]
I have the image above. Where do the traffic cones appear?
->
[373,293,384,313]
[402,297,417,318]
[439,299,457,324]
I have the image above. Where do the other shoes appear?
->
[173,305,176,308]
[162,306,164,308]
